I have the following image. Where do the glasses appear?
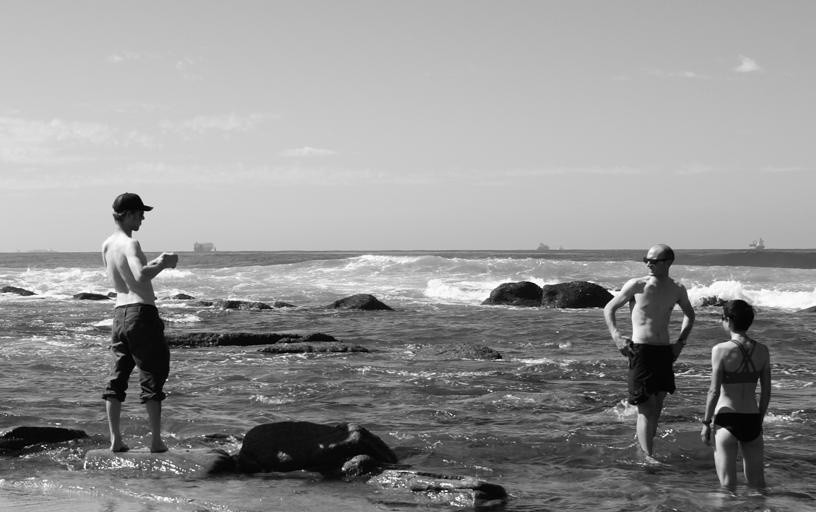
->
[643,257,670,264]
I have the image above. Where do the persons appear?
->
[700,299,773,493]
[101,191,177,453]
[602,244,694,458]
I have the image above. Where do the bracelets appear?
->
[702,417,713,425]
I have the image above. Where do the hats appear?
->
[114,193,153,213]
